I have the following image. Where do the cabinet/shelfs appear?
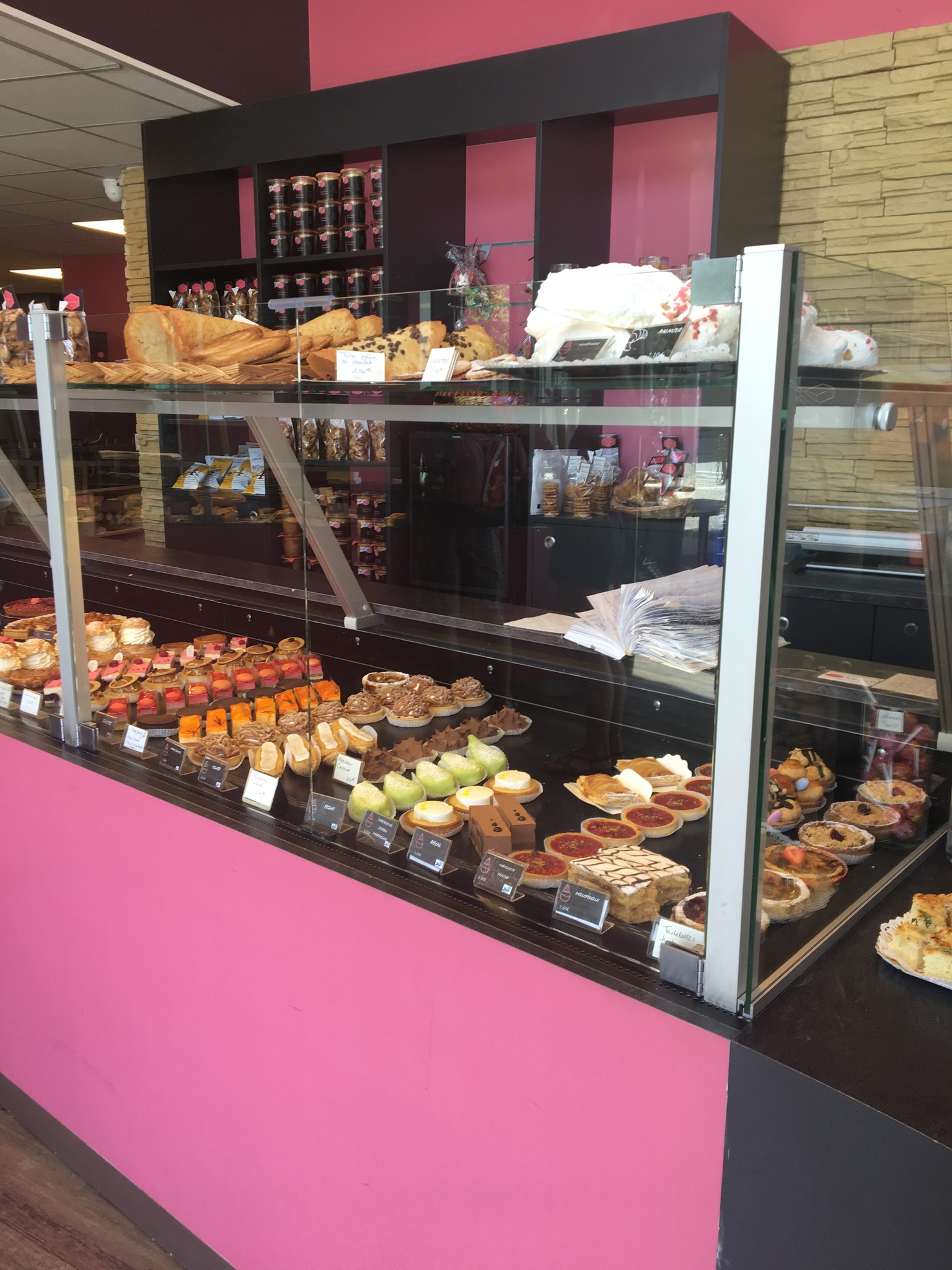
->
[139,8,935,672]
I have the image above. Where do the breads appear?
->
[74,487,276,523]
[123,304,528,380]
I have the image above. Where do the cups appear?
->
[267,206,290,233]
[551,264,579,272]
[355,542,387,585]
[356,519,386,543]
[281,493,303,571]
[370,220,383,248]
[345,268,372,296]
[290,203,317,230]
[315,199,341,227]
[290,176,318,203]
[370,266,383,294]
[316,228,341,254]
[290,230,318,256]
[315,172,340,200]
[268,232,290,258]
[342,225,369,252]
[271,272,318,298]
[342,197,368,224]
[638,252,710,281]
[340,168,366,197]
[266,179,291,205]
[356,496,386,520]
[312,489,350,561]
[320,271,343,298]
[369,192,382,220]
[370,164,383,193]
[272,296,384,331]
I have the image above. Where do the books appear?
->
[564,561,793,673]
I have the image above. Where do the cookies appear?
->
[173,287,258,324]
[0,308,90,367]
[542,467,676,518]
[278,420,385,462]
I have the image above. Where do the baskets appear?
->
[610,466,695,519]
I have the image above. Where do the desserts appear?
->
[760,747,952,982]
[0,596,712,933]
